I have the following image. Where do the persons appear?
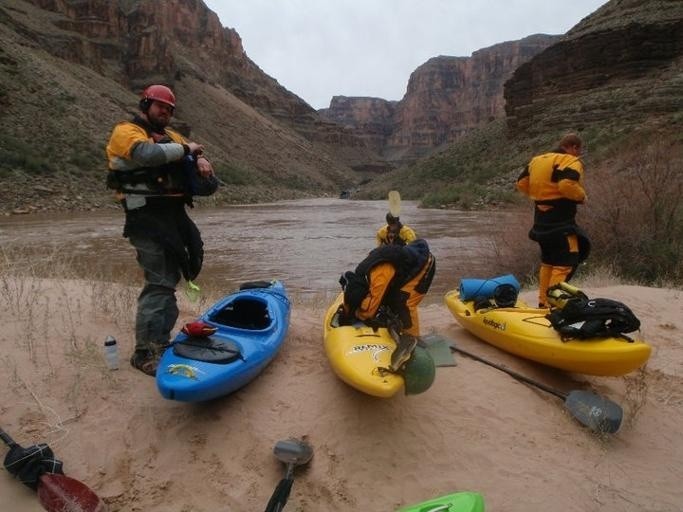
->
[106,83,216,378]
[342,238,435,339]
[376,209,416,247]
[517,135,592,307]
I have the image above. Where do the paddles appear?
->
[450,346,623,434]
[264,439,314,512]
[0,429,99,511]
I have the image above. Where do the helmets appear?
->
[494,283,516,307]
[386,212,400,224]
[140,84,176,107]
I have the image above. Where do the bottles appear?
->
[103,335,120,371]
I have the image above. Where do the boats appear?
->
[322,290,406,399]
[443,288,652,377]
[156,278,291,404]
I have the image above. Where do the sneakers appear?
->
[130,346,170,377]
